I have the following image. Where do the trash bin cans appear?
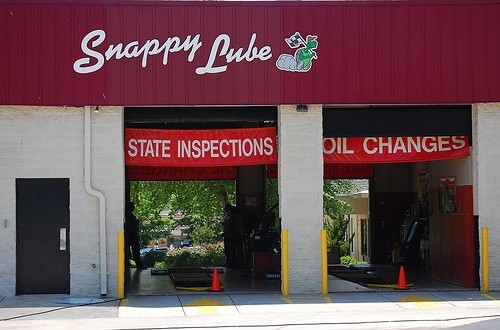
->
[155,247,167,263]
[327,246,341,264]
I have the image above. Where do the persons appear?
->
[126,202,147,269]
[221,202,246,269]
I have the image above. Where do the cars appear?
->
[180,238,192,247]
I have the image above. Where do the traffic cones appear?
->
[395,266,410,290]
[209,268,224,291]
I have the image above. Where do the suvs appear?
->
[139,247,169,267]
